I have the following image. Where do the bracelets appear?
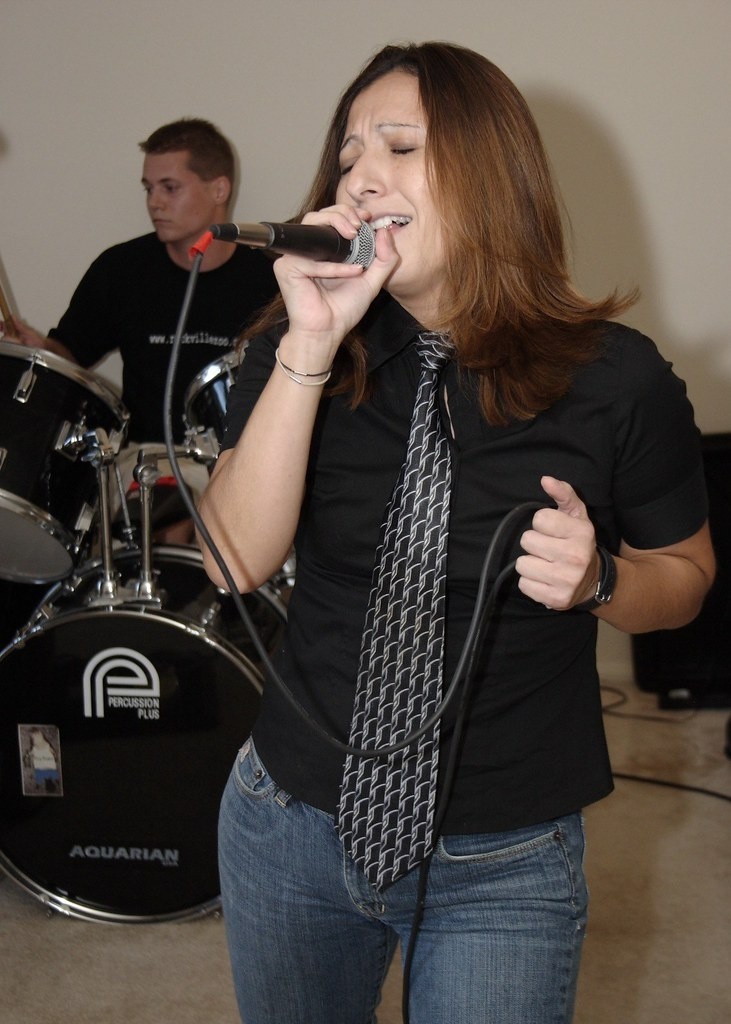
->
[574,542,617,612]
[275,347,331,386]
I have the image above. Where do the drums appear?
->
[0,340,128,585]
[182,343,247,462]
[0,540,288,929]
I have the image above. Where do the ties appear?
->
[337,329,460,895]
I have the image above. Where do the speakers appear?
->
[629,432,731,710]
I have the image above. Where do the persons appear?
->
[193,41,717,1024]
[0,118,279,545]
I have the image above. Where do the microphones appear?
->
[211,219,376,270]
